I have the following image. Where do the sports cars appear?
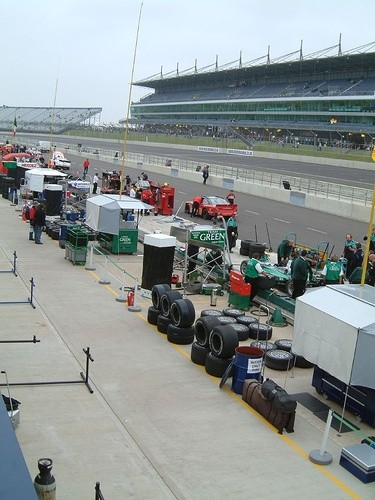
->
[185,196,238,220]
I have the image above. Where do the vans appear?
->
[36,140,51,152]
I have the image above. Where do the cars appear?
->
[50,152,71,170]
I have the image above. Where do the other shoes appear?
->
[35,241,43,244]
[29,238,35,240]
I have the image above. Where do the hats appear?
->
[31,201,40,206]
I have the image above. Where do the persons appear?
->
[206,249,223,271]
[124,172,158,215]
[92,173,99,194]
[226,212,238,253]
[188,246,199,272]
[343,234,356,261]
[289,250,313,299]
[226,190,235,204]
[202,166,209,184]
[369,227,375,250]
[348,267,369,284]
[29,201,40,241]
[245,253,272,302]
[369,254,375,285]
[191,196,202,217]
[348,242,364,274]
[277,240,294,266]
[5,139,26,153]
[33,204,46,244]
[83,159,89,173]
[321,255,343,284]
